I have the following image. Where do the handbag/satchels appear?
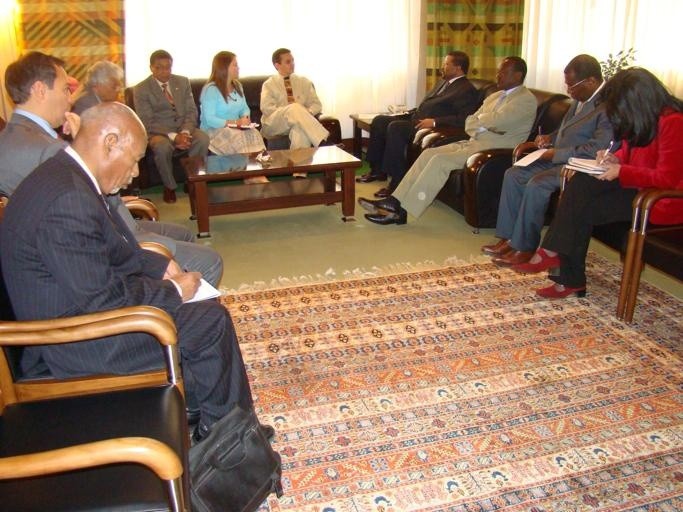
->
[186,407,285,511]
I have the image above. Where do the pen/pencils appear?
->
[599,141,614,165]
[539,126,543,136]
[183,264,189,272]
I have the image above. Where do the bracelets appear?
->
[433,121,436,128]
[235,120,236,124]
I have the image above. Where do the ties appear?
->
[161,84,175,107]
[444,81,450,89]
[496,94,506,110]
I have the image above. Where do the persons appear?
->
[510,65,682,299]
[1,100,276,449]
[355,50,481,198]
[63,60,151,203]
[260,48,345,180]
[358,56,538,225]
[1,51,225,290]
[198,49,274,184]
[132,50,210,205]
[481,54,621,266]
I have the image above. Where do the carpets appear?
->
[219,249,683,512]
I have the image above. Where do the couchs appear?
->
[125,76,341,189]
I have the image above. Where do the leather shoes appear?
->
[356,172,407,225]
[192,424,274,446]
[482,239,534,265]
[164,187,176,203]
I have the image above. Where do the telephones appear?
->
[388,104,407,114]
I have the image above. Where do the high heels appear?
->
[513,249,561,276]
[536,283,586,299]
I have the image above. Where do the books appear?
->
[228,122,260,129]
[513,148,549,167]
[563,154,608,175]
[184,278,221,303]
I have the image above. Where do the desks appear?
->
[349,111,391,158]
